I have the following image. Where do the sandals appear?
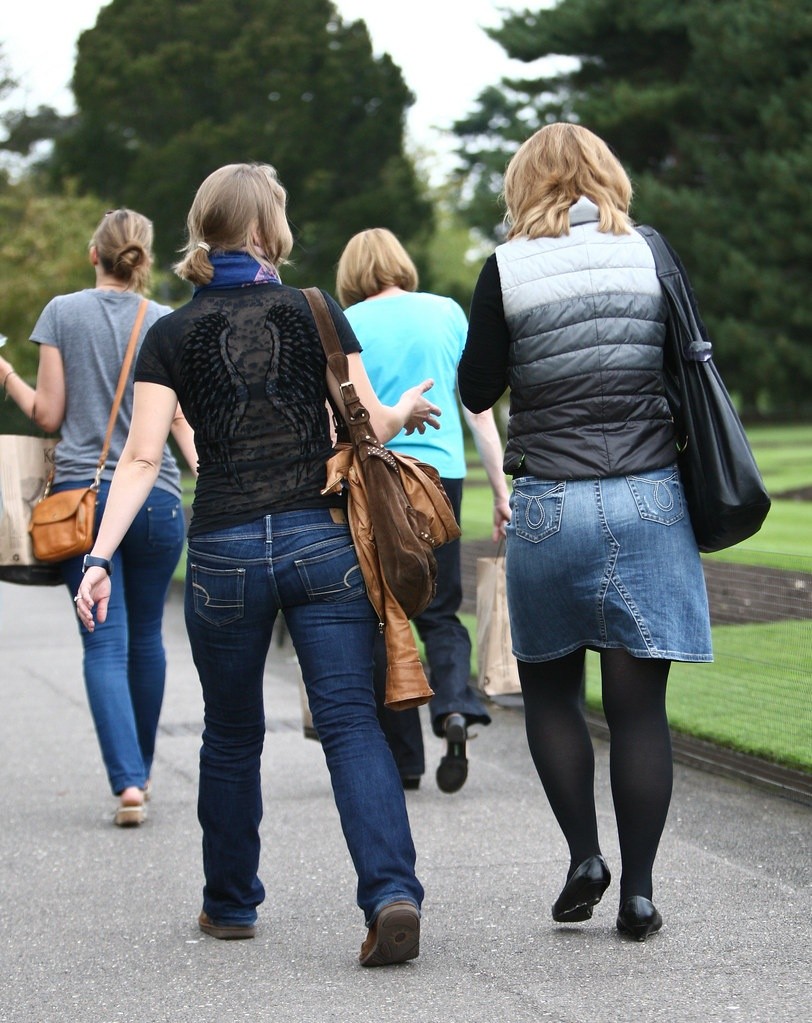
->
[118,784,151,824]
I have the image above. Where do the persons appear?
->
[0,208,201,826]
[458,121,715,942]
[323,227,514,794]
[77,161,441,965]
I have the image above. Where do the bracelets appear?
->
[4,372,17,402]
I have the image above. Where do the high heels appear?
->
[552,856,609,923]
[615,896,663,941]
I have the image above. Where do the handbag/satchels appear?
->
[634,224,772,554]
[299,665,314,739]
[346,403,438,620]
[28,486,96,561]
[476,535,523,696]
[0,391,61,565]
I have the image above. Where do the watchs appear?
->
[81,554,113,575]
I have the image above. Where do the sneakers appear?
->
[197,909,255,941]
[360,901,421,966]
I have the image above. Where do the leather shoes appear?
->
[399,773,421,790]
[435,714,468,793]
